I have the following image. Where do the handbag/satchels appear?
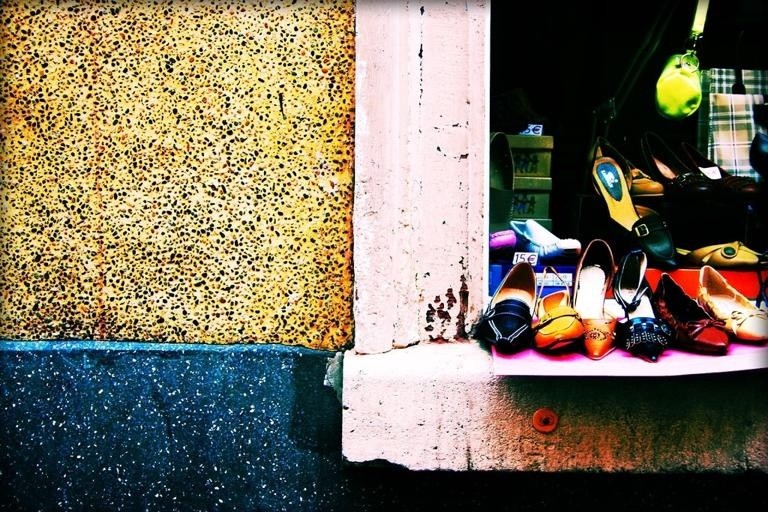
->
[655,52,703,119]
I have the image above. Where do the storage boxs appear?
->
[512,178,551,220]
[503,135,552,176]
[514,220,552,235]
